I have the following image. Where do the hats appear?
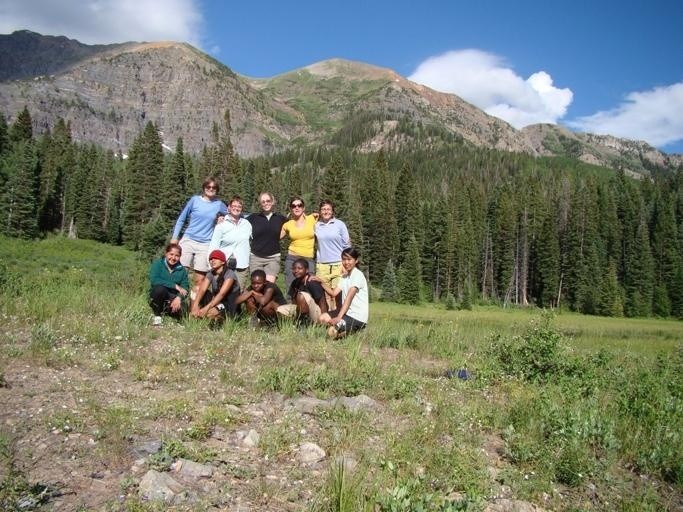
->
[210,251,226,261]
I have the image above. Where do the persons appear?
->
[308,248,369,340]
[217,191,319,284]
[147,243,190,326]
[235,270,288,325]
[314,200,351,311]
[191,250,241,320]
[275,259,329,324]
[209,196,253,294]
[279,197,319,302]
[170,176,228,312]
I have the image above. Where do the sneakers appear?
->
[153,315,161,324]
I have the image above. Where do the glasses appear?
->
[206,185,217,190]
[292,203,303,208]
